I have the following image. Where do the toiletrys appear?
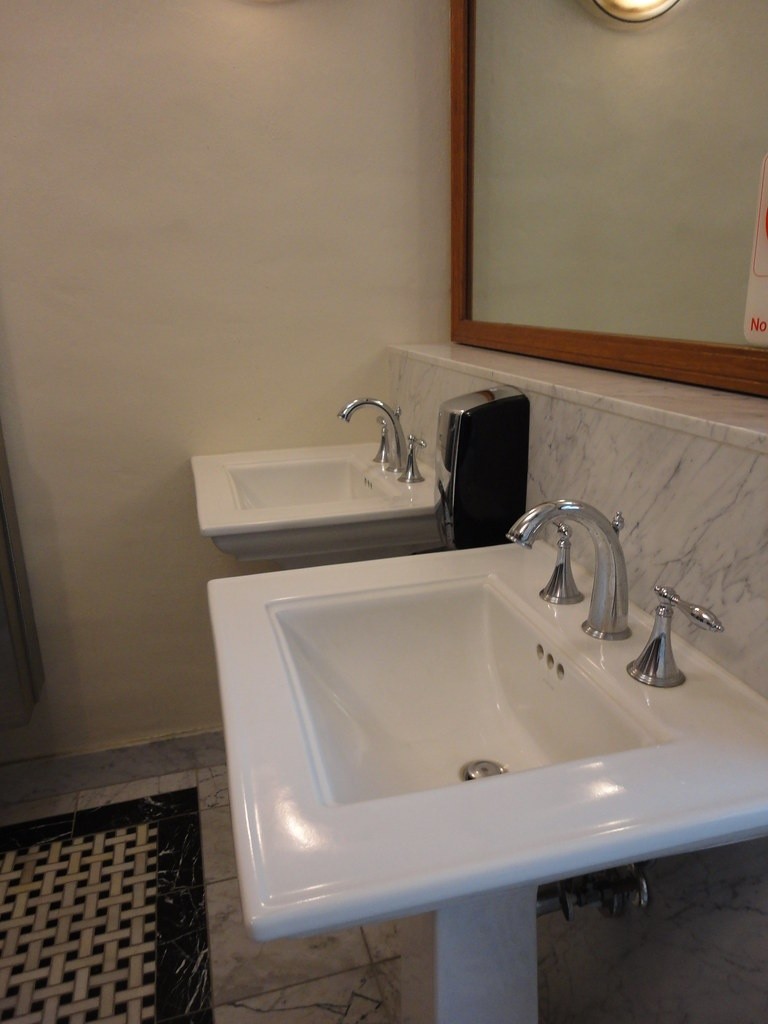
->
[427,381,529,553]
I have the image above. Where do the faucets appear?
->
[334,395,411,474]
[504,496,640,646]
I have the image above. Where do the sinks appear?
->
[188,439,442,568]
[204,538,768,957]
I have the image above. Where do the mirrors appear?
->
[467,0,767,350]
